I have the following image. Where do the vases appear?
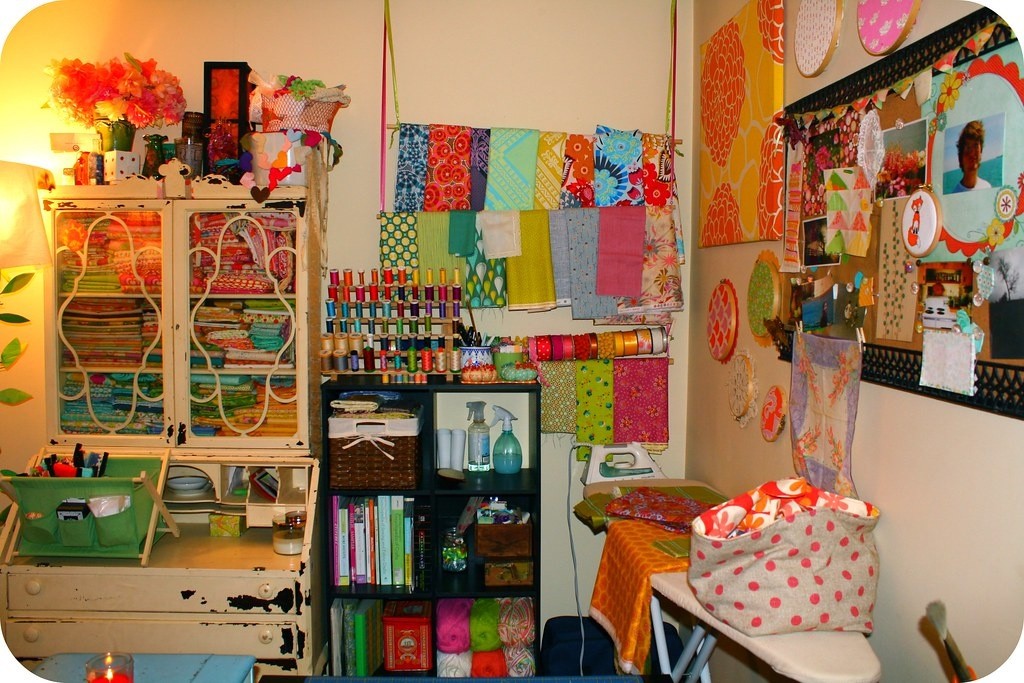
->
[94,118,135,151]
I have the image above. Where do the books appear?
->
[331,494,431,677]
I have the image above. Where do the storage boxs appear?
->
[329,405,424,487]
[484,557,534,586]
[382,601,432,671]
[202,61,256,175]
[208,513,249,538]
[475,519,532,556]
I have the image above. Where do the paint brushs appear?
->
[918,600,976,683]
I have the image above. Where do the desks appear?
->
[583,479,882,683]
[31,654,257,683]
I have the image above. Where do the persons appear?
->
[819,302,831,328]
[952,120,992,195]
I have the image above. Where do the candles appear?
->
[88,669,132,683]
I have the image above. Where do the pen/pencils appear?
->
[40,443,109,479]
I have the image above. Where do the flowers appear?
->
[40,51,186,130]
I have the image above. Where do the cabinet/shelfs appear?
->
[321,379,543,679]
[320,279,463,380]
[0,157,329,682]
